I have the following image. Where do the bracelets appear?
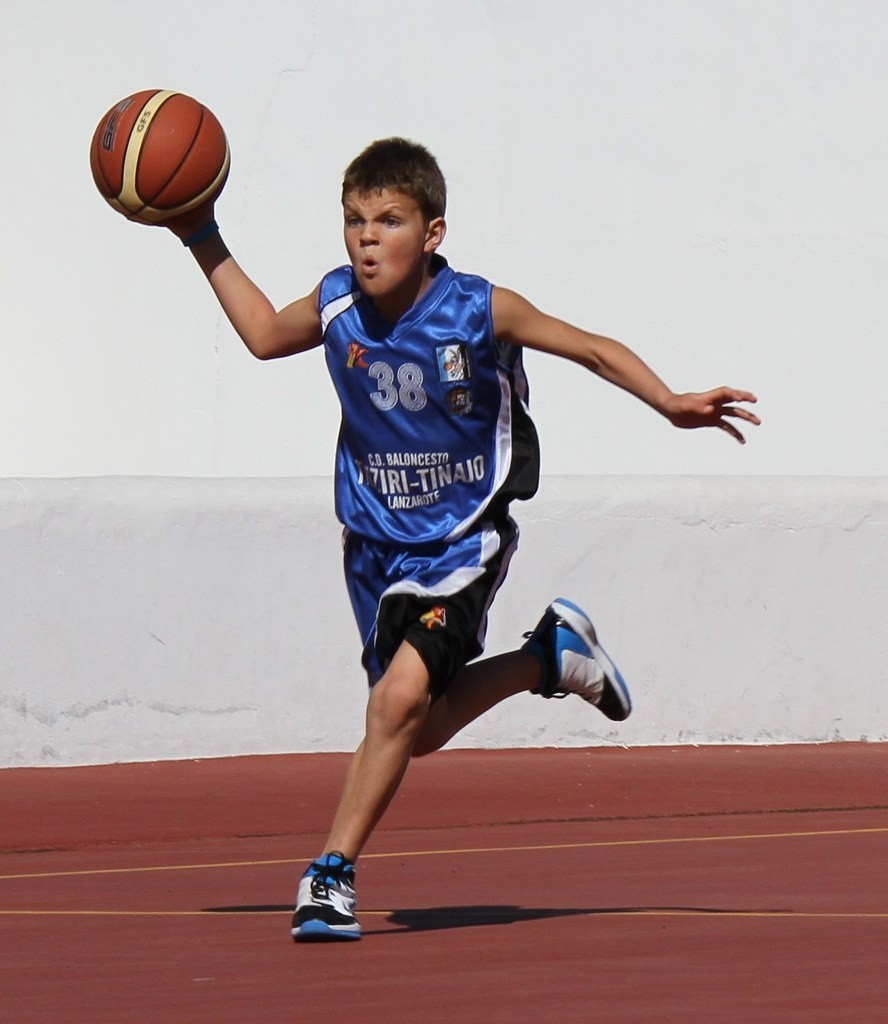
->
[183,222,218,248]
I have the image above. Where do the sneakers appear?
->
[523,597,631,722]
[291,850,362,939]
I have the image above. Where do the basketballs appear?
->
[88,89,229,225]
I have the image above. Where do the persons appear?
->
[150,142,762,942]
